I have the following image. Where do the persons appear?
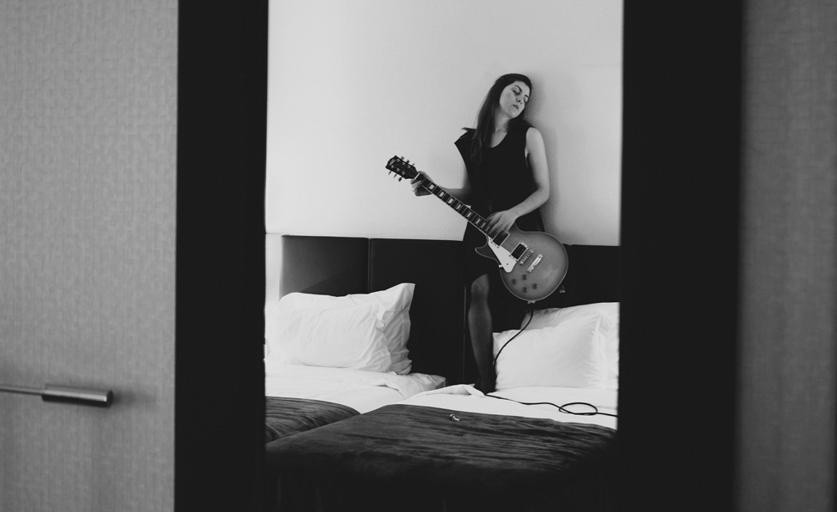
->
[411,73,551,395]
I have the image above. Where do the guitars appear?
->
[386,155,569,303]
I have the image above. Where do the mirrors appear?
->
[169,2,746,511]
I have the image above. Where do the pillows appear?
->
[481,324,621,394]
[512,298,620,331]
[266,281,417,376]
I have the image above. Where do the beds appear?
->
[263,380,621,512]
[228,367,447,447]
